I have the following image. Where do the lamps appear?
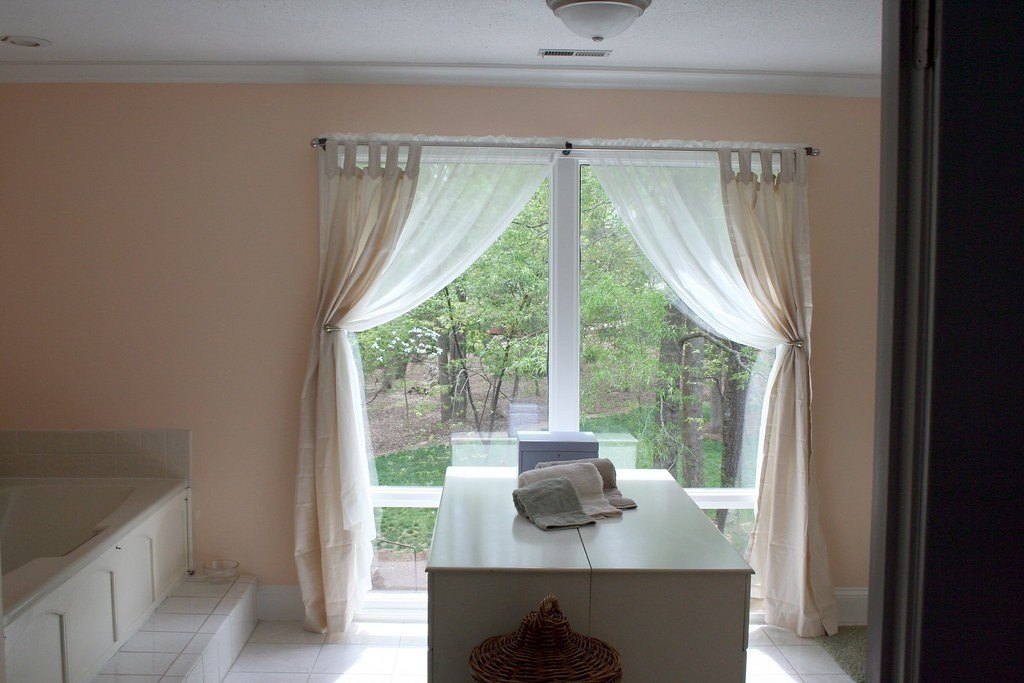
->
[546,0,652,42]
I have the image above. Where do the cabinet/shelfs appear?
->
[424,465,755,683]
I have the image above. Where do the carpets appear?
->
[814,625,865,683]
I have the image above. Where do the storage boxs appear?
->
[516,430,600,476]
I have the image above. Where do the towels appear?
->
[512,458,637,531]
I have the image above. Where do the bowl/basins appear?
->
[203,559,240,585]
